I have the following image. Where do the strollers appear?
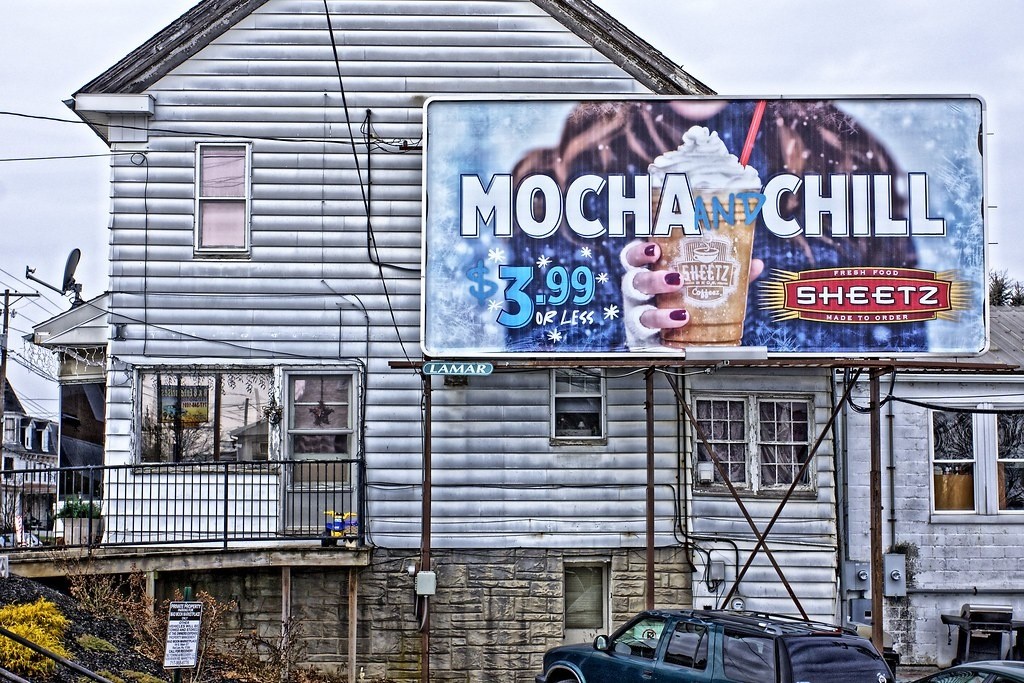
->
[319,509,359,550]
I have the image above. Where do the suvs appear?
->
[533,608,896,683]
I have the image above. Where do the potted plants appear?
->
[58,493,101,550]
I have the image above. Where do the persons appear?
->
[505,100,931,350]
